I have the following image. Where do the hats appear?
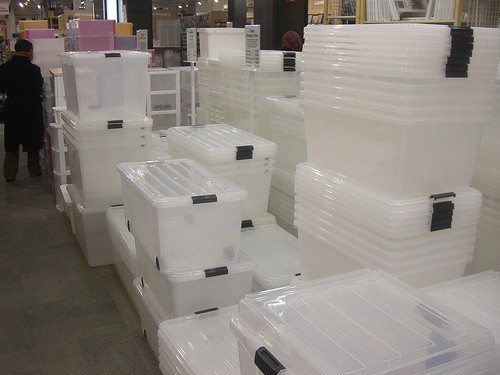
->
[284,31,302,51]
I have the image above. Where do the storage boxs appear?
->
[15,8,500,375]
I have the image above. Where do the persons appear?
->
[0,39,46,183]
[278,31,302,71]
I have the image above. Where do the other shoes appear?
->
[31,169,41,177]
[7,179,14,181]
